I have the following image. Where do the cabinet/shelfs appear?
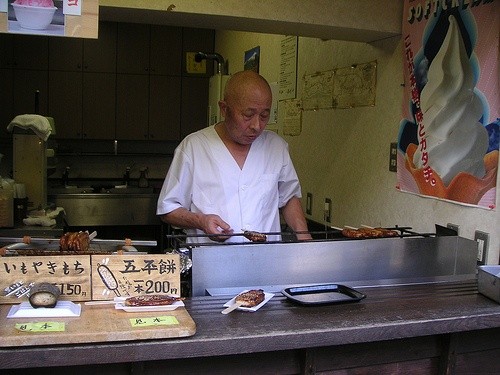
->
[0,21,215,141]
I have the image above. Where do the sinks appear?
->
[46,177,172,228]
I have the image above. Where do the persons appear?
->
[156,70,312,246]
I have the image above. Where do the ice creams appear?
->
[399,0,500,207]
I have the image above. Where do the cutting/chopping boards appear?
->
[0,302,196,350]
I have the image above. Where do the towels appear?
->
[7,114,53,142]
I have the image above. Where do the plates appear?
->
[282,284,367,307]
[223,290,275,311]
[6,301,82,319]
[111,297,186,313]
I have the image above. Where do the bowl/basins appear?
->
[477,264,500,304]
[10,3,58,29]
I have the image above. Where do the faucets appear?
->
[138,165,150,188]
